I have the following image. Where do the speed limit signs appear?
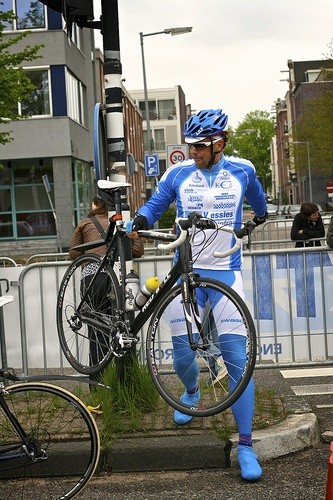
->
[168,151,185,165]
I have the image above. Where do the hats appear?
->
[185,136,206,143]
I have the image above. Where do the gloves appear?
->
[132,214,149,233]
[253,212,269,227]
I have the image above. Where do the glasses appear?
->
[186,137,223,148]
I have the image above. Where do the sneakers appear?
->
[237,445,262,480]
[173,388,200,425]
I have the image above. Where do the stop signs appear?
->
[326,182,333,193]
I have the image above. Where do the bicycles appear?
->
[56,178,258,419]
[0,366,112,500]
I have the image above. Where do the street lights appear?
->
[289,141,312,203]
[138,25,194,230]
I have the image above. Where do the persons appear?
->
[291,202,326,248]
[69,191,146,393]
[125,108,267,480]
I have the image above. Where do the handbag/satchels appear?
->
[113,235,132,261]
[96,189,115,207]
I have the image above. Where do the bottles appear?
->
[133,276,159,310]
[125,270,140,311]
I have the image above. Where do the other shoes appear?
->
[207,354,228,386]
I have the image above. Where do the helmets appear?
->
[183,109,229,143]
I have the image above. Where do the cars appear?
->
[266,204,301,218]
